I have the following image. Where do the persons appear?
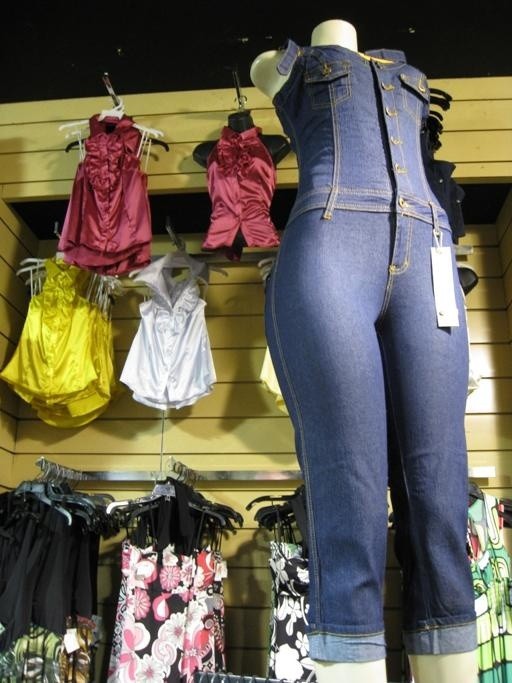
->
[252,20,481,681]
[193,110,290,248]
